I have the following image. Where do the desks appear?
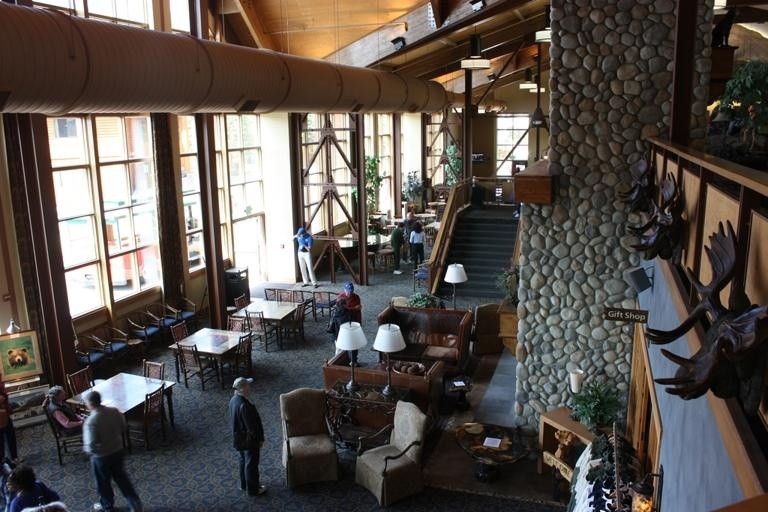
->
[337,200,446,275]
[325,379,414,450]
[456,423,531,484]
[374,359,433,376]
[537,406,597,484]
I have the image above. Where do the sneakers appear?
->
[93,503,113,512]
[393,270,401,274]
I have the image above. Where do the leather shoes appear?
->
[256,485,266,495]
[314,285,316,288]
[301,284,308,287]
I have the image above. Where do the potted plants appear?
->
[720,62,768,172]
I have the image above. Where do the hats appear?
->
[232,377,253,390]
[344,283,353,291]
[297,227,304,234]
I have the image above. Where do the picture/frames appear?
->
[0,329,44,382]
[7,384,49,430]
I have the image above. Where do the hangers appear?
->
[607,431,626,511]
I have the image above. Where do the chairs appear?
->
[354,399,429,506]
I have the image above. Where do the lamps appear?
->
[518,66,546,93]
[531,43,547,127]
[443,262,468,311]
[372,323,407,396]
[534,3,552,42]
[451,107,487,114]
[335,322,368,391]
[460,26,490,70]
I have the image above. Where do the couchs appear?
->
[322,348,444,411]
[377,305,473,376]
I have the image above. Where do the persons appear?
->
[80,392,142,512]
[401,211,422,261]
[1,449,69,512]
[229,377,266,496]
[327,296,357,365]
[292,227,318,288]
[48,386,85,440]
[391,223,406,274]
[1,370,18,466]
[409,222,427,272]
[336,282,363,324]
